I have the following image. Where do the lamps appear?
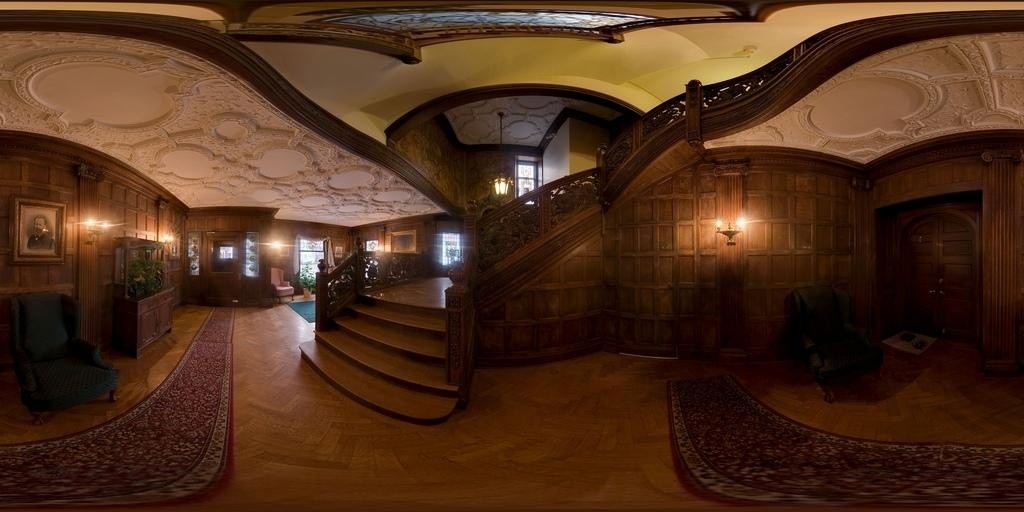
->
[487,112,514,199]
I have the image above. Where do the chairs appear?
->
[8,291,119,424]
[269,268,294,304]
[789,284,884,386]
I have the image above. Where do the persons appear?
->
[27,215,55,251]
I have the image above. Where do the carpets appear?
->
[286,300,316,323]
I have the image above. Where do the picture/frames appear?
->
[6,192,68,265]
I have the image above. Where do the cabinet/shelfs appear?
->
[109,237,177,359]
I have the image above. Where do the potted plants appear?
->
[294,263,316,298]
